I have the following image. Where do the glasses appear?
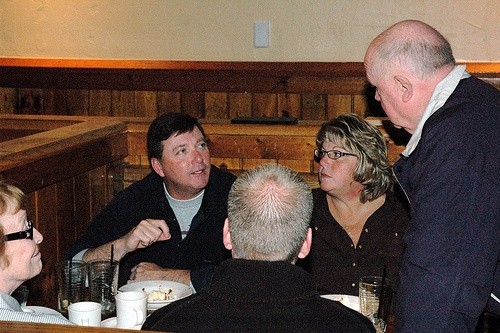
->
[314,148,357,160]
[3,220,34,241]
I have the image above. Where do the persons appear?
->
[292,112,410,322]
[0,175,76,325]
[141,162,378,333]
[63,112,238,295]
[364,20,500,332]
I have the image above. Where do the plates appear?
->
[321,295,359,312]
[101,316,117,329]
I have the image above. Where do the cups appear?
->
[11,285,30,307]
[115,291,145,331]
[87,258,118,316]
[68,301,102,328]
[55,260,87,313]
[359,275,386,333]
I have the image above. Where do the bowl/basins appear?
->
[118,281,192,311]
